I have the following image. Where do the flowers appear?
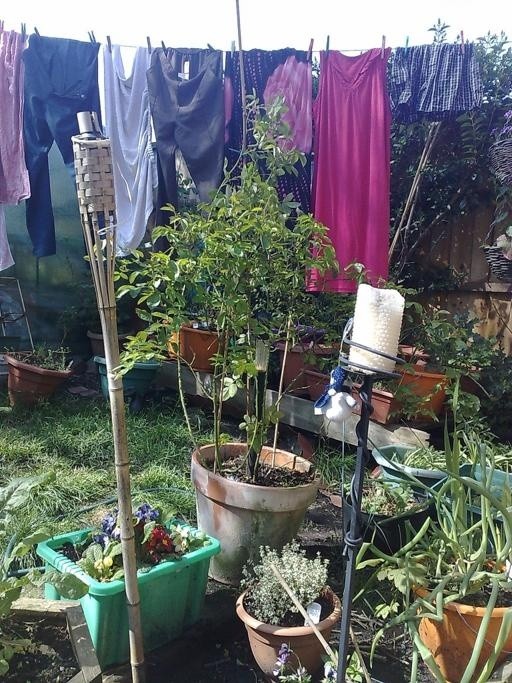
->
[78,498,208,584]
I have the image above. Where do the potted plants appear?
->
[86,82,511,681]
[0,517,107,683]
[2,347,75,417]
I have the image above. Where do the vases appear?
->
[36,518,221,673]
